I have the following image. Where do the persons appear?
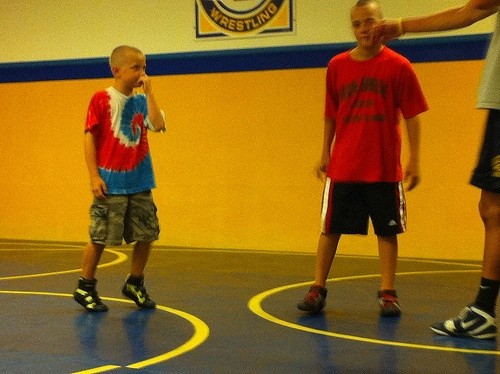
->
[296,0,429,317]
[73,45,166,313]
[369,0,500,340]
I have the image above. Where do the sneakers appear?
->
[297,284,328,312]
[122,273,155,309]
[378,290,402,316]
[74,277,108,312]
[429,303,497,338]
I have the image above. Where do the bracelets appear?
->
[397,17,404,35]
